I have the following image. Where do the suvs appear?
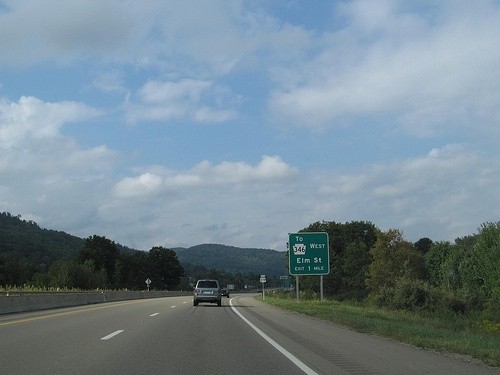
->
[193,279,221,307]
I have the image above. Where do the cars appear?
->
[257,289,262,293]
[222,289,229,298]
